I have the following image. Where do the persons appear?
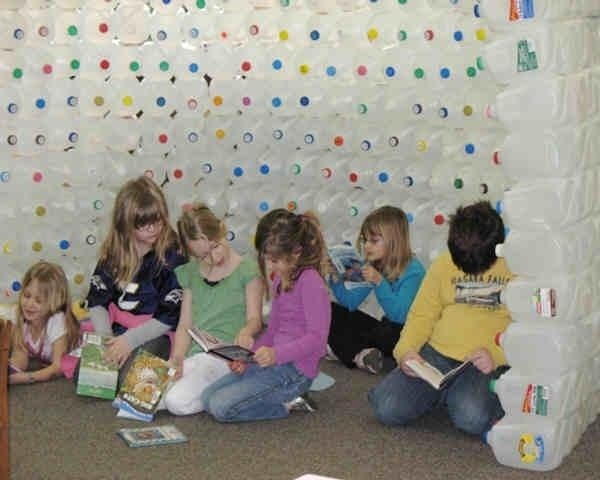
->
[343,265,353,281]
[327,205,426,375]
[200,208,332,423]
[153,202,264,417]
[350,259,363,282]
[365,200,517,436]
[84,175,189,392]
[7,261,81,386]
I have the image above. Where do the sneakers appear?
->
[286,394,316,412]
[353,348,381,374]
[324,343,338,361]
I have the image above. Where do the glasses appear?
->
[133,216,163,231]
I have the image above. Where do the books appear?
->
[326,244,376,291]
[111,348,178,423]
[117,424,189,448]
[186,327,257,364]
[75,331,120,401]
[404,358,474,391]
